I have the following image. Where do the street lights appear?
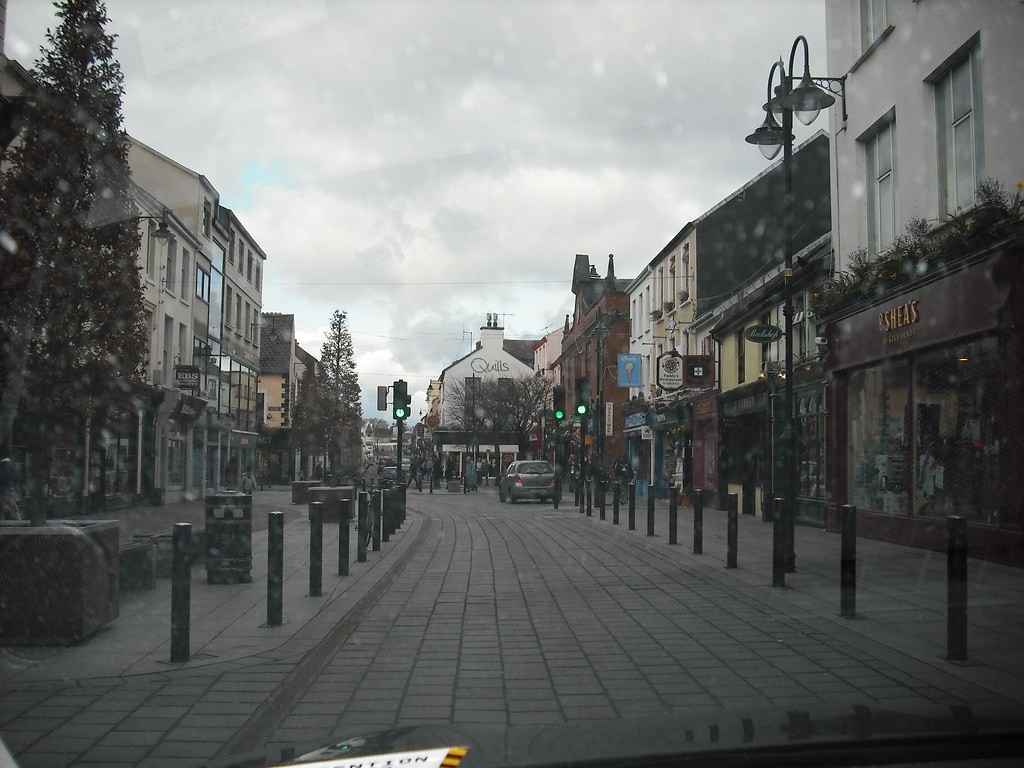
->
[575,377,590,416]
[745,35,835,573]
[591,316,609,508]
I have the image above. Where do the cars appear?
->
[498,460,562,504]
[380,467,406,483]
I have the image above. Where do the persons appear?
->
[226,460,234,490]
[584,454,593,480]
[0,444,21,520]
[316,464,322,480]
[241,467,256,495]
[568,458,579,493]
[407,459,418,490]
[613,455,633,502]
[417,455,490,492]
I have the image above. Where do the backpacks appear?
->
[618,461,629,478]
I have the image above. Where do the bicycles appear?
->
[600,471,626,506]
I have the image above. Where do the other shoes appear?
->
[467,490,470,492]
[476,488,477,492]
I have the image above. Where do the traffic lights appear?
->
[553,386,567,422]
[393,379,411,420]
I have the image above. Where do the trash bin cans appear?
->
[206,491,253,584]
